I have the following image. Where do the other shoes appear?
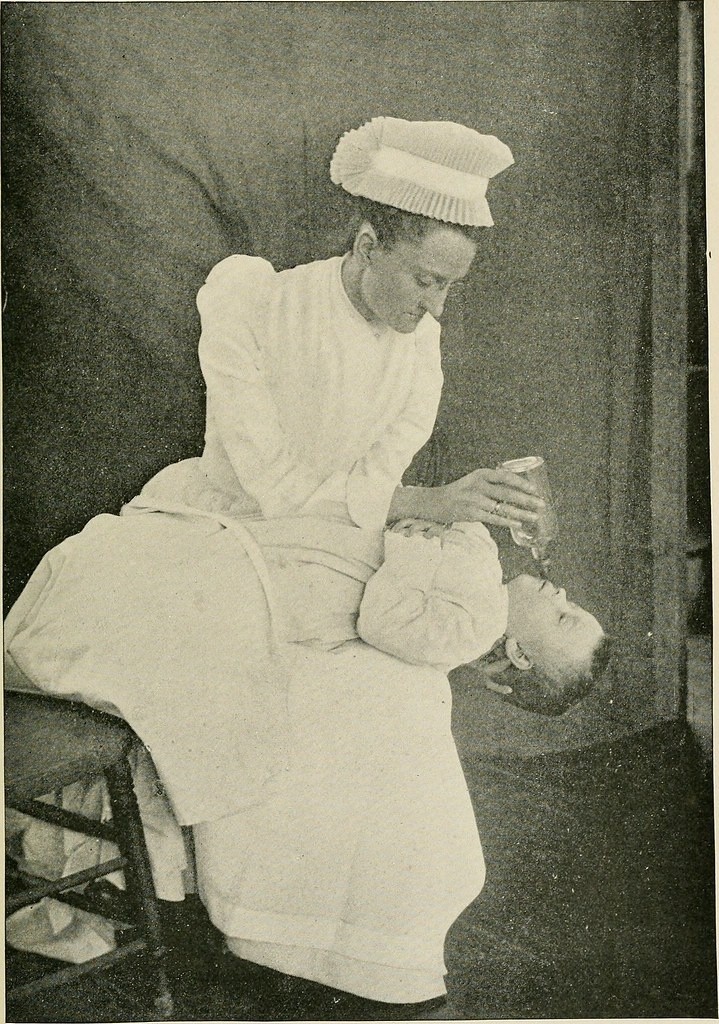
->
[319,985,448,1020]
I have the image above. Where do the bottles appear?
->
[495,455,561,581]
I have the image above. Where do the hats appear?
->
[329,117,515,227]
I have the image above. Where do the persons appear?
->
[3,114,545,1005]
[240,509,611,718]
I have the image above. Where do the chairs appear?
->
[5,687,177,1023]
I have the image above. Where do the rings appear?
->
[492,501,501,514]
